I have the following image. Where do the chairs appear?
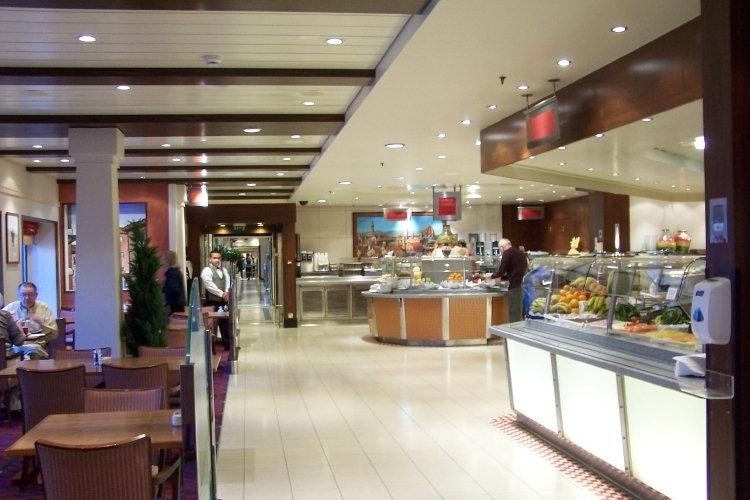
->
[32,431,183,500]
[78,384,167,413]
[15,362,90,435]
[2,283,228,398]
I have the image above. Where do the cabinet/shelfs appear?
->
[523,255,707,365]
[352,284,372,320]
[299,286,351,320]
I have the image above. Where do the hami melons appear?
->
[607,269,630,294]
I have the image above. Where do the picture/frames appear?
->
[6,211,21,265]
[61,199,150,294]
[352,211,447,257]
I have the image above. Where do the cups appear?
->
[413,272,421,284]
[21,321,28,334]
[641,231,658,252]
[579,301,589,316]
[343,264,362,276]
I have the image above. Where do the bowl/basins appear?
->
[442,250,451,257]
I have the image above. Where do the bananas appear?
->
[584,295,605,313]
[530,297,546,314]
[550,302,569,314]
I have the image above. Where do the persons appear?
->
[200,250,231,351]
[519,245,533,276]
[492,238,526,320]
[245,254,252,280]
[1,282,59,361]
[0,292,26,359]
[466,237,476,255]
[186,246,194,295]
[161,250,186,313]
[457,239,476,275]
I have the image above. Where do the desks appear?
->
[2,408,187,471]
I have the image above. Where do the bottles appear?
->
[218,305,229,315]
[675,230,691,254]
[92,346,102,367]
[657,229,676,254]
[313,252,330,272]
